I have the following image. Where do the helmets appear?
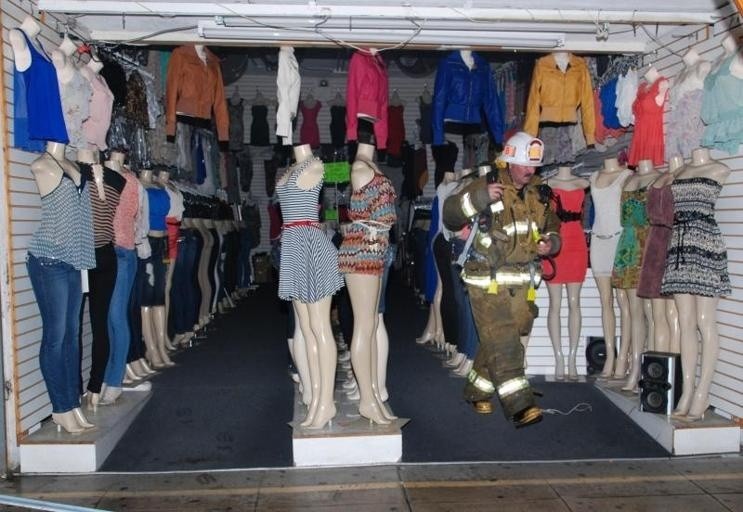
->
[499,132,544,168]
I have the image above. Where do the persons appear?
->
[6,16,260,438]
[259,44,743,174]
[274,139,401,431]
[409,148,735,425]
[444,132,564,427]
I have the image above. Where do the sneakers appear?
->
[472,400,493,414]
[512,406,542,428]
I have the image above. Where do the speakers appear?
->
[640,351,682,417]
[585,336,621,377]
